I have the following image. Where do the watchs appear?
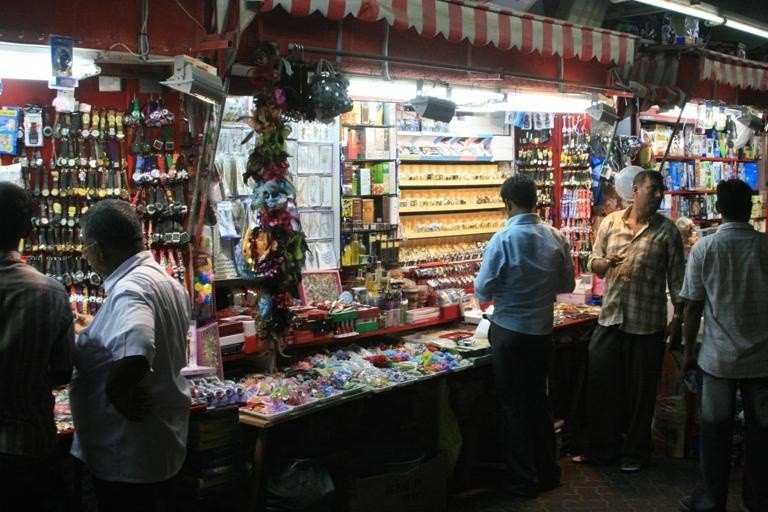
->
[15,96,194,318]
[184,373,247,410]
[514,114,591,224]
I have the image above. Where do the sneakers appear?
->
[570,452,645,473]
[679,493,691,511]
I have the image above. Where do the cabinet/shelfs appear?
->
[209,77,768,351]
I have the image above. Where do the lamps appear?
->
[637,0,768,39]
[159,51,223,107]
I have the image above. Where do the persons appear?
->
[679,177,768,511]
[471,172,578,500]
[65,195,195,512]
[567,169,688,473]
[0,182,78,512]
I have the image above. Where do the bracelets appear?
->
[674,312,684,320]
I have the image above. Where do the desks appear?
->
[53,292,600,512]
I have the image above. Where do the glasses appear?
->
[80,241,97,255]
